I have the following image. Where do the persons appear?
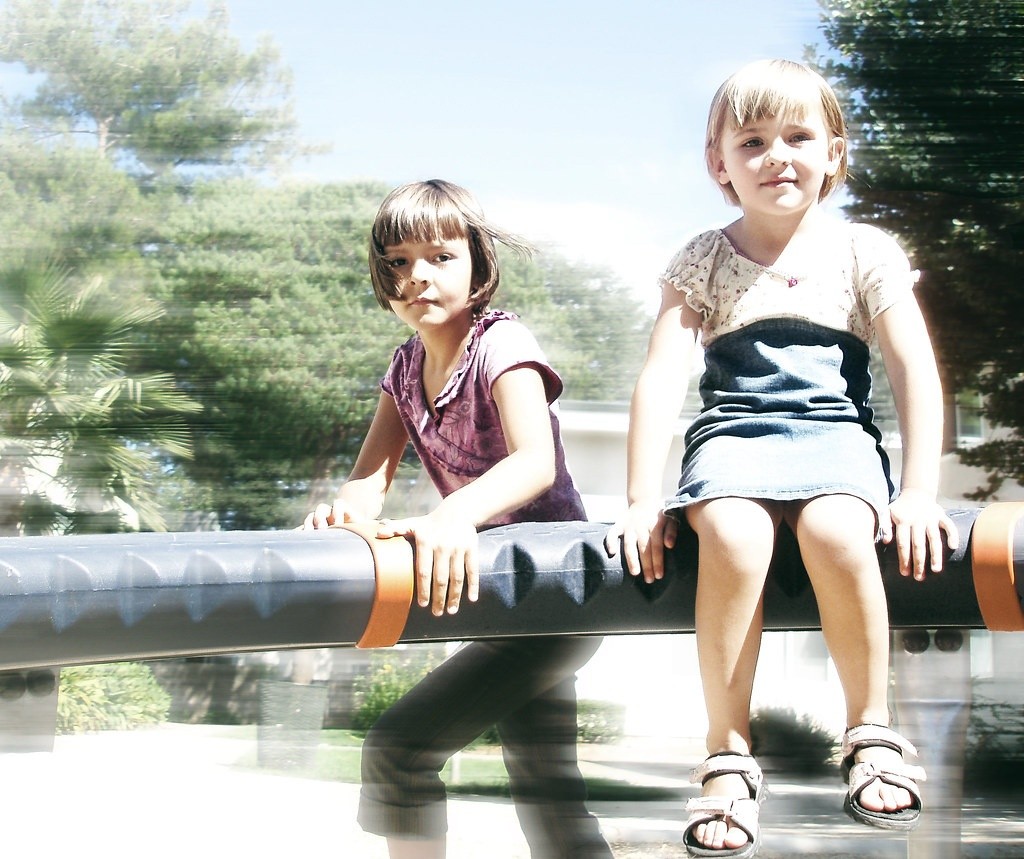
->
[604,59,960,859]
[289,178,622,859]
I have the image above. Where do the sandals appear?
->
[840,722,926,831]
[682,750,764,859]
